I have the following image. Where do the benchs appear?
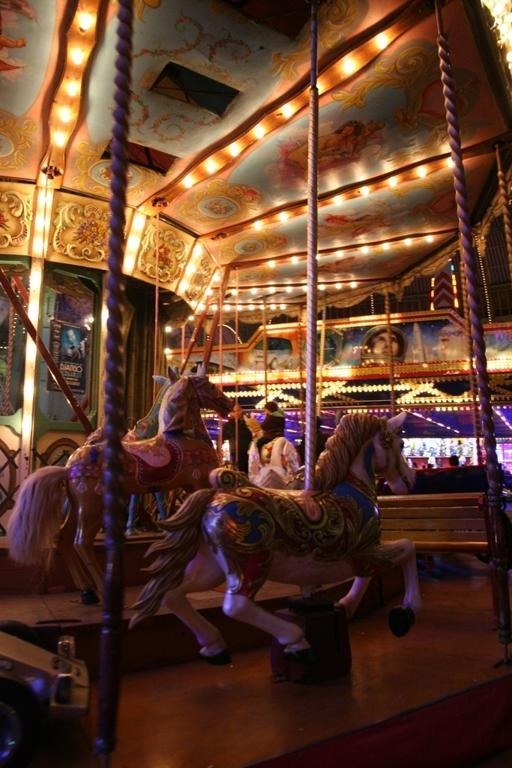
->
[375,491,489,551]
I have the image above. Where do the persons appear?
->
[66,329,85,361]
[294,416,330,466]
[215,404,253,475]
[256,402,285,457]
[67,346,75,358]
[449,455,459,467]
